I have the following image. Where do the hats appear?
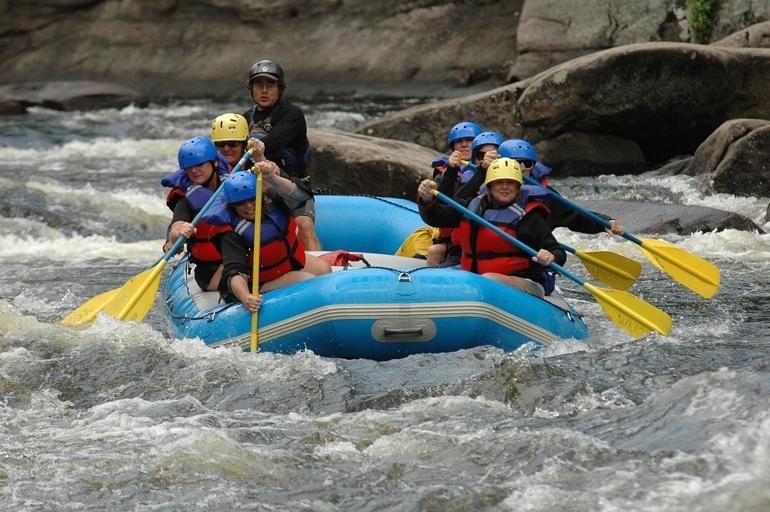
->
[251,72,278,82]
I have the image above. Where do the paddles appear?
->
[522,173,721,299]
[430,187,672,340]
[458,161,641,292]
[103,148,255,323]
[60,236,187,326]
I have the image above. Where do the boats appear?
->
[160,193,590,362]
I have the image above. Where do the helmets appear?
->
[448,121,481,143]
[248,60,285,82]
[224,171,257,204]
[470,131,502,161]
[210,113,250,143]
[485,158,523,185]
[498,138,536,167]
[178,136,219,170]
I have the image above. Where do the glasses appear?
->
[215,141,243,148]
[514,160,534,170]
[475,149,486,160]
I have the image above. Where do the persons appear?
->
[159,59,333,315]
[415,121,622,292]
